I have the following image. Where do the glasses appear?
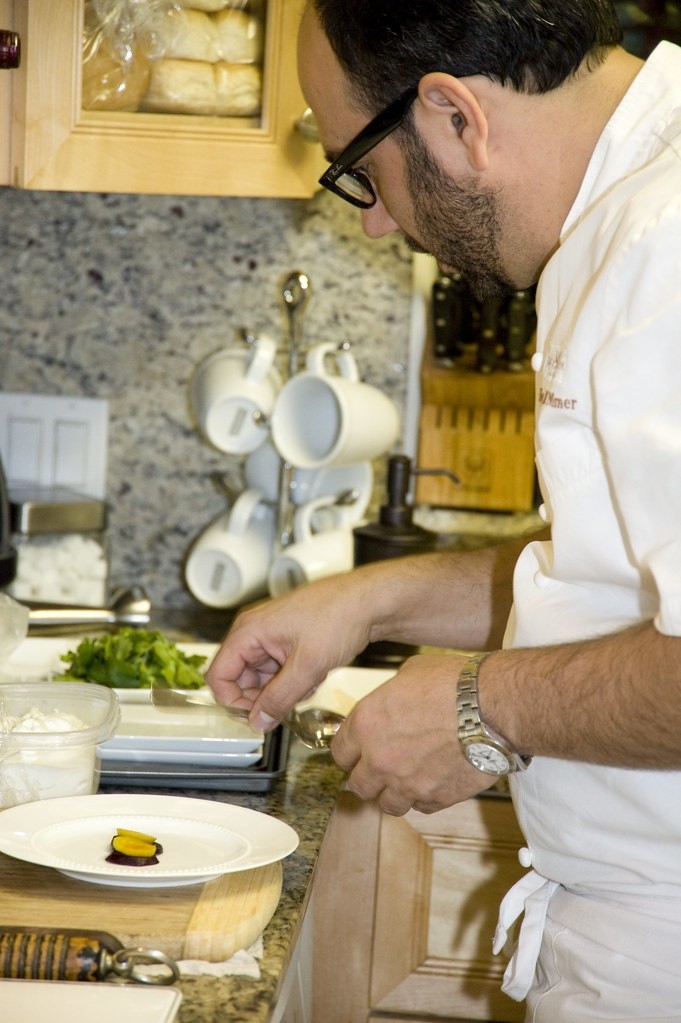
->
[315,65,485,210]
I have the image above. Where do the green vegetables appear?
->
[53,626,208,689]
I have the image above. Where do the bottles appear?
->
[353,454,460,673]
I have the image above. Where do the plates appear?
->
[97,687,266,767]
[0,979,183,1023]
[0,793,300,888]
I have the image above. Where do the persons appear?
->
[210,1,677,1023]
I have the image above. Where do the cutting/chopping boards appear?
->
[0,854,285,963]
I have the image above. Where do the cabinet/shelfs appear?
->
[370,796,531,1023]
[3,2,317,200]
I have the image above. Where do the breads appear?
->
[82,1,263,119]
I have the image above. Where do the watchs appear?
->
[456,653,533,777]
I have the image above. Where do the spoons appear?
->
[150,682,347,749]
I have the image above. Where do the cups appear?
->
[270,342,400,469]
[187,333,283,456]
[268,495,369,600]
[183,486,286,610]
[242,438,375,519]
